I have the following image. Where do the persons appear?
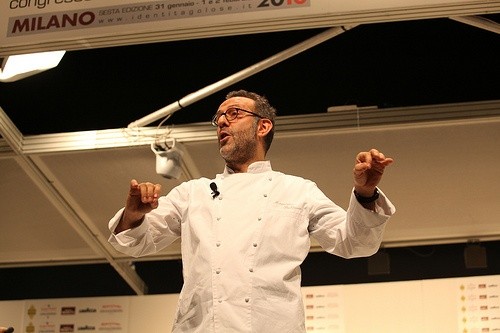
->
[107,91,396,333]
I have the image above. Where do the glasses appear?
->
[210,107,268,126]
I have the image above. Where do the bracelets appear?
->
[354,188,380,204]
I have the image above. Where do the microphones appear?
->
[210,182,220,198]
